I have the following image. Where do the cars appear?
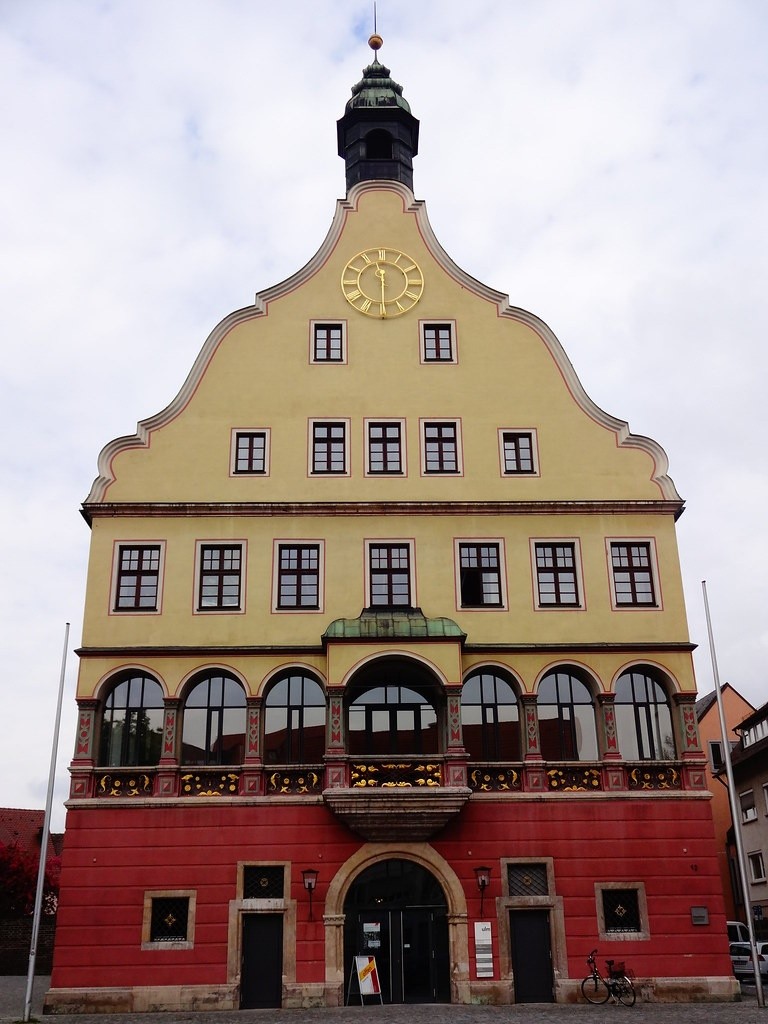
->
[726,921,750,943]
[729,941,768,984]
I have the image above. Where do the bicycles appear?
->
[578,950,637,1006]
